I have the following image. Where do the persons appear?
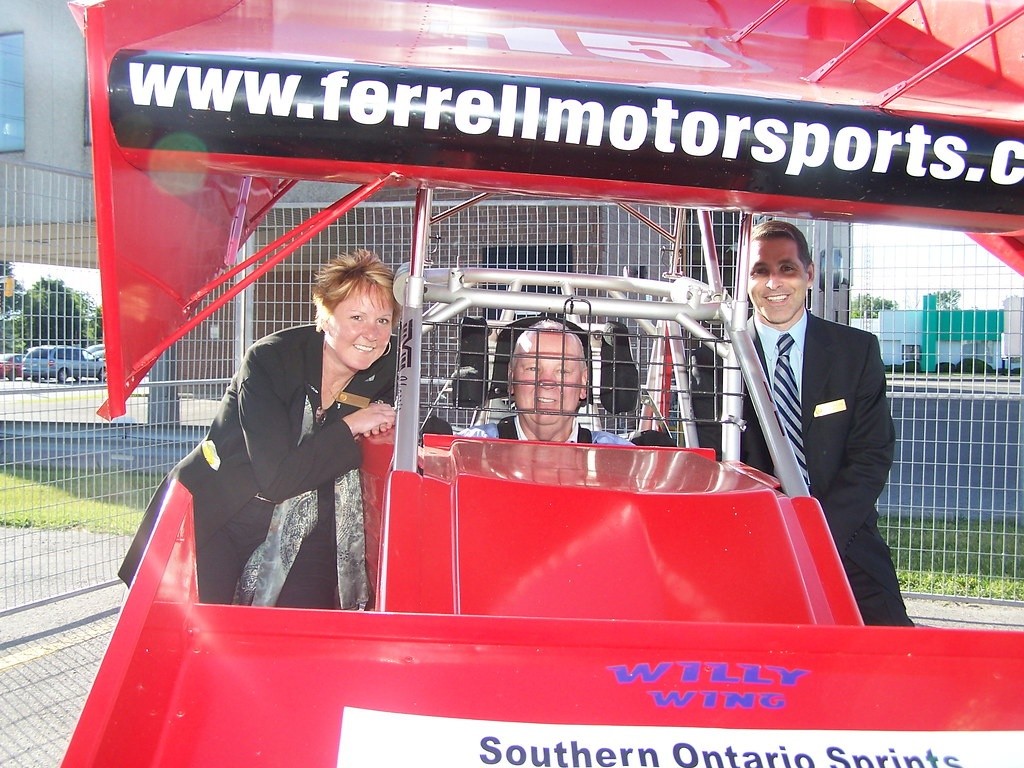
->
[118,251,400,613]
[691,221,916,626]
[458,320,634,448]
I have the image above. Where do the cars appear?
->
[0,353,24,381]
[86,344,104,362]
[21,344,106,383]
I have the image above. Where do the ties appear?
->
[772,334,809,490]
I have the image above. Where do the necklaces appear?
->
[315,375,347,427]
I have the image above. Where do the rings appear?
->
[376,399,384,404]
